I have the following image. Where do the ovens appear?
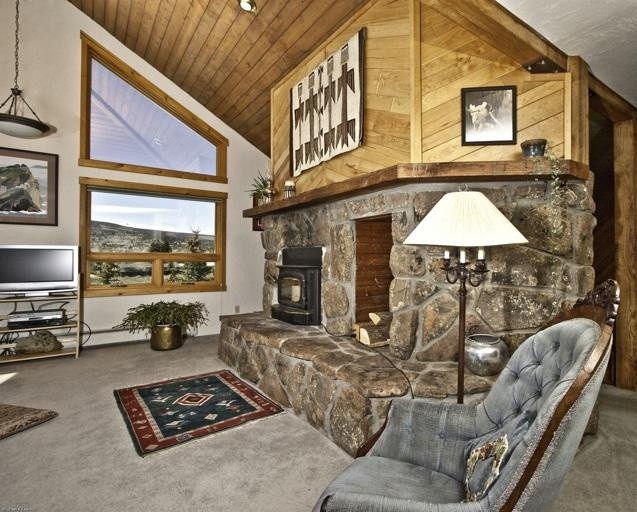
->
[271,247,322,325]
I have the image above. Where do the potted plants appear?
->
[110,298,210,351]
[244,169,268,207]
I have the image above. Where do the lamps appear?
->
[0,0,50,140]
[401,191,529,404]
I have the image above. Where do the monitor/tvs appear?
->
[1,245,77,296]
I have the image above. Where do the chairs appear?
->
[310,278,621,512]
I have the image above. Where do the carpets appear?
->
[114,370,285,459]
[0,403,60,440]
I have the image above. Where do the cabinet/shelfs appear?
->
[0,272,82,364]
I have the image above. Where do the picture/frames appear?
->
[0,146,58,226]
[460,85,517,146]
